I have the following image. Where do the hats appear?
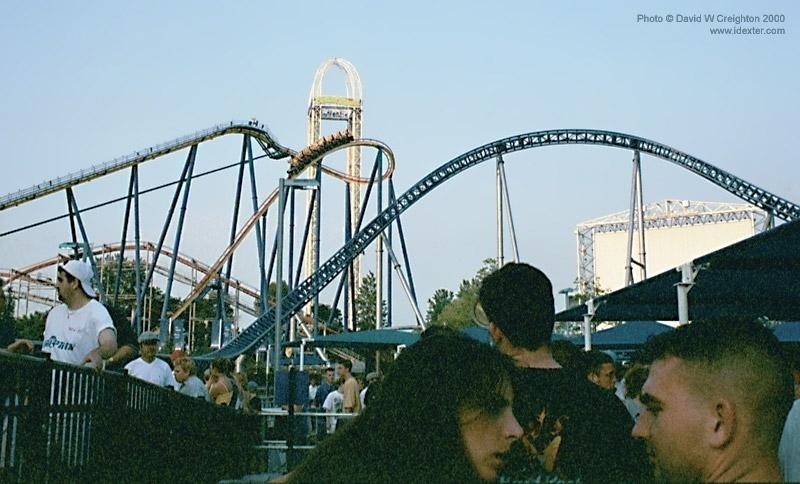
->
[138,332,160,344]
[247,382,258,390]
[366,373,379,382]
[59,260,97,298]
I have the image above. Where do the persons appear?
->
[0,288,32,434]
[265,260,800,484]
[45,260,260,483]
